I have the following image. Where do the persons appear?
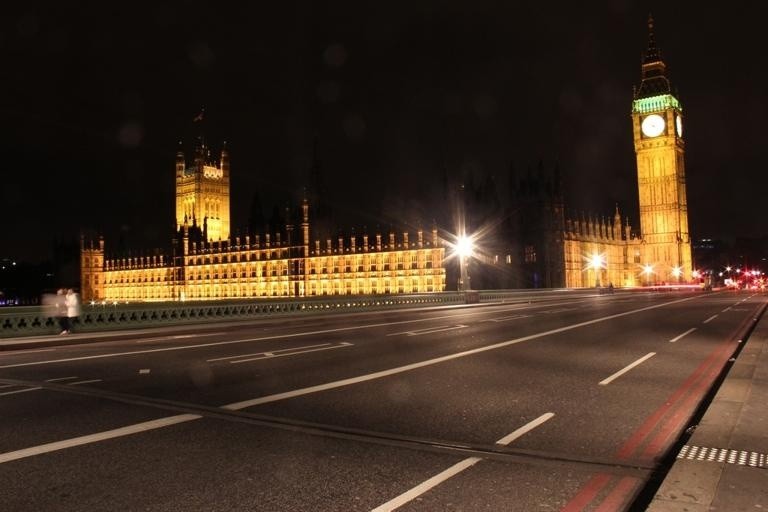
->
[62,287,82,334]
[54,287,68,336]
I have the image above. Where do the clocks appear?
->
[641,114,683,137]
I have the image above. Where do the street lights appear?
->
[453,235,473,289]
[591,255,602,287]
[644,266,652,286]
